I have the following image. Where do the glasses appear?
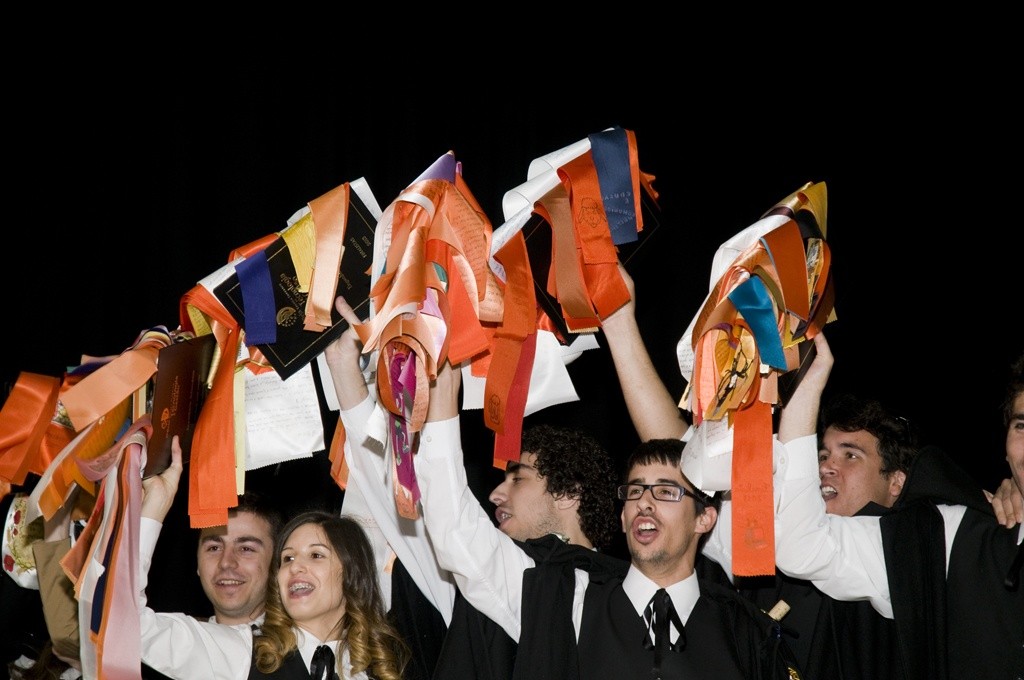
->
[617,484,706,506]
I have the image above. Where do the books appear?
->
[214,185,377,382]
[522,182,664,346]
[139,334,217,480]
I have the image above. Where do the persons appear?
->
[324,261,1024,680]
[0,434,412,680]
